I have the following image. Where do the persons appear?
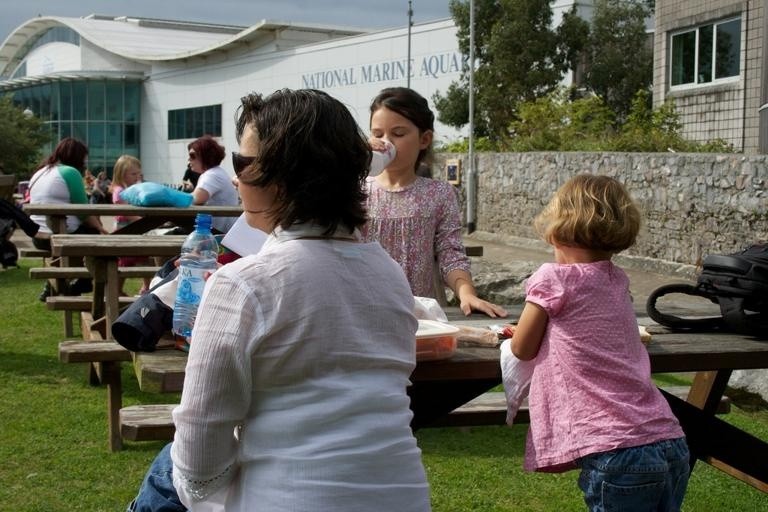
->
[0,136,238,266]
[498,174,690,510]
[358,88,509,318]
[126,86,431,512]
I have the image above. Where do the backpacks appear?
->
[646,243,768,340]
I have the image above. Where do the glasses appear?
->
[232,152,257,177]
[189,151,197,160]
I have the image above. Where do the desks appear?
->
[129,322,768,496]
[22,203,488,451]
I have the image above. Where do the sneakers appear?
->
[39,282,58,303]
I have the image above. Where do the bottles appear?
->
[173,212,220,336]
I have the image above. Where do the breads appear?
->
[457,326,499,348]
[638,326,651,342]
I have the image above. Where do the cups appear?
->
[367,140,397,178]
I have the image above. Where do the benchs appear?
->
[20,249,730,451]
